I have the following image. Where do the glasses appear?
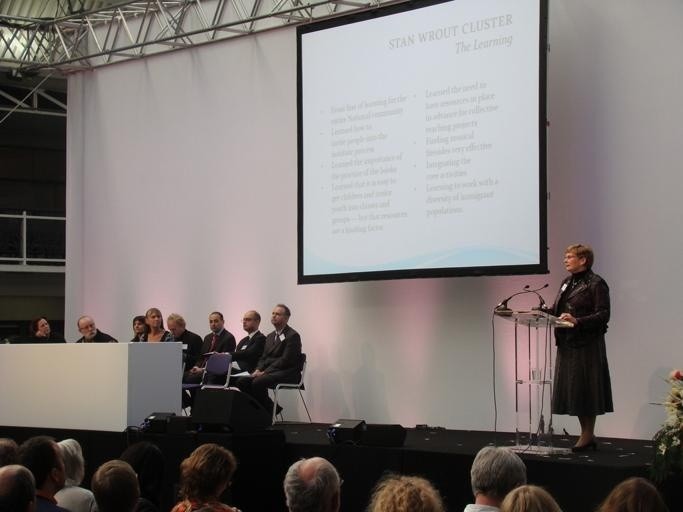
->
[79,322,94,332]
[562,255,576,259]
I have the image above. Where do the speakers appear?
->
[191,388,270,432]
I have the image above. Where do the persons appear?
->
[234,303,302,413]
[463,446,563,512]
[30,315,65,342]
[601,478,667,512]
[166,313,205,411]
[75,315,119,343]
[128,315,146,341]
[212,310,265,386]
[0,436,241,512]
[284,456,342,512]
[182,311,238,414]
[136,308,173,342]
[368,476,443,512]
[547,244,614,454]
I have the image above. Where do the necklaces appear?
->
[571,278,581,289]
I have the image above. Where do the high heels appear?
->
[571,439,597,453]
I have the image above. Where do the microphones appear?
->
[522,285,554,314]
[494,283,549,315]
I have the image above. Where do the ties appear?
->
[200,335,216,368]
[274,334,280,344]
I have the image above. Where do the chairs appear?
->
[182,350,315,428]
